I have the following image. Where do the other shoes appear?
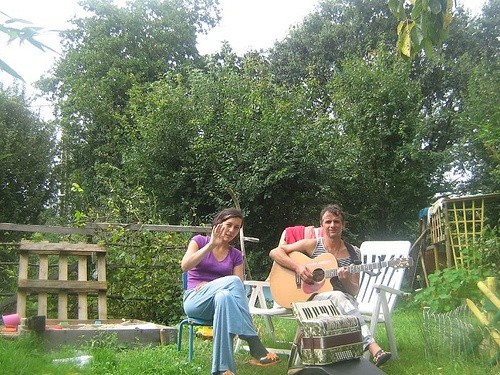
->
[372,350,392,367]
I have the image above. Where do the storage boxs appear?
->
[290,300,364,365]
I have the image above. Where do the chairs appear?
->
[176,272,215,361]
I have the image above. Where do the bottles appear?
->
[159,328,167,346]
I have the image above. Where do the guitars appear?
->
[267,250,414,311]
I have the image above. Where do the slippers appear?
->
[248,352,283,366]
[220,370,234,375]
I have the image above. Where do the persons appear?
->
[269,202,392,367]
[181,208,282,375]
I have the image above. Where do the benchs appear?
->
[234,226,412,365]
